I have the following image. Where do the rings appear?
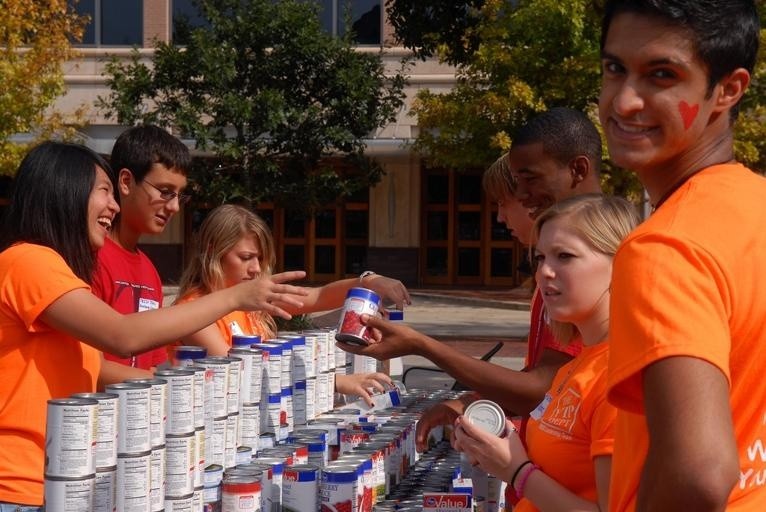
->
[509,427,518,433]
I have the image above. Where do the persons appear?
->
[167,203,412,409]
[83,123,195,370]
[334,106,603,512]
[453,194,642,512]
[482,153,553,247]
[0,140,310,512]
[598,2,765,512]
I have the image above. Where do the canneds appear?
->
[222,386,505,511]
[232,324,335,444]
[336,348,377,377]
[43,347,263,512]
[333,286,383,347]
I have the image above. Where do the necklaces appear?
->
[557,338,607,394]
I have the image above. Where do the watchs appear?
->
[359,271,377,287]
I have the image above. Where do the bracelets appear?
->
[510,460,536,489]
[517,464,544,500]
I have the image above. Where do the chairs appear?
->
[403,342,504,390]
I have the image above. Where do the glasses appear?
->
[142,177,192,204]
